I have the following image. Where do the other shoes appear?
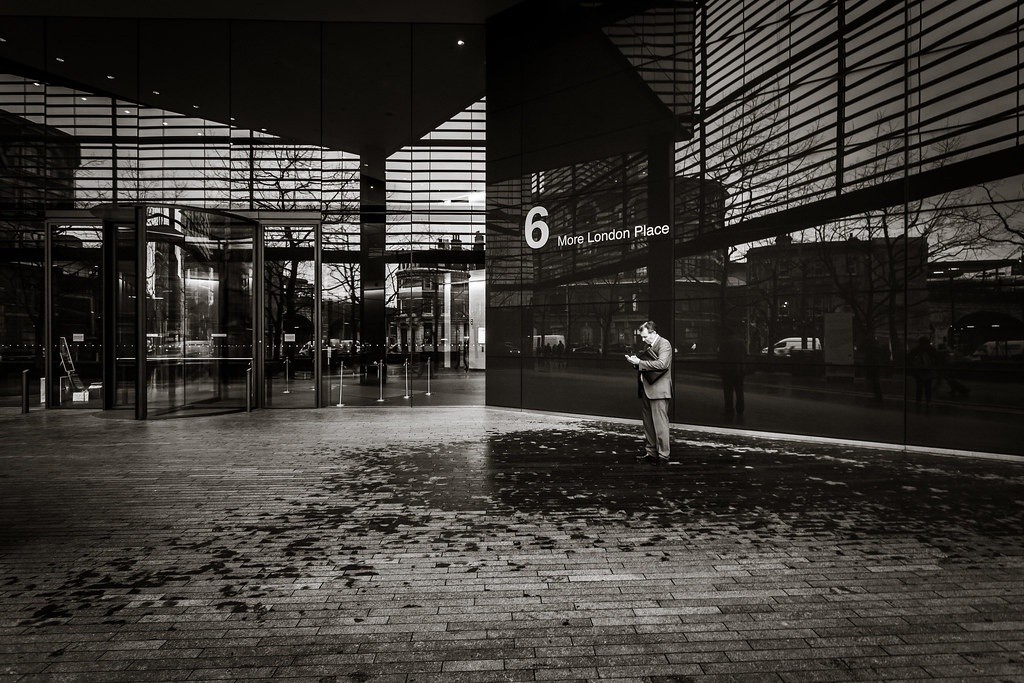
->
[656,457,667,466]
[636,453,651,461]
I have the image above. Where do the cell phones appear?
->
[625,355,630,359]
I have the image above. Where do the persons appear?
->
[536,340,569,369]
[719,329,746,418]
[626,321,672,463]
[351,344,357,352]
[907,337,939,415]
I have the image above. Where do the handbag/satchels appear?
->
[635,347,669,386]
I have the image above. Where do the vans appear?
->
[760,336,823,358]
[532,334,566,354]
[970,339,1024,362]
